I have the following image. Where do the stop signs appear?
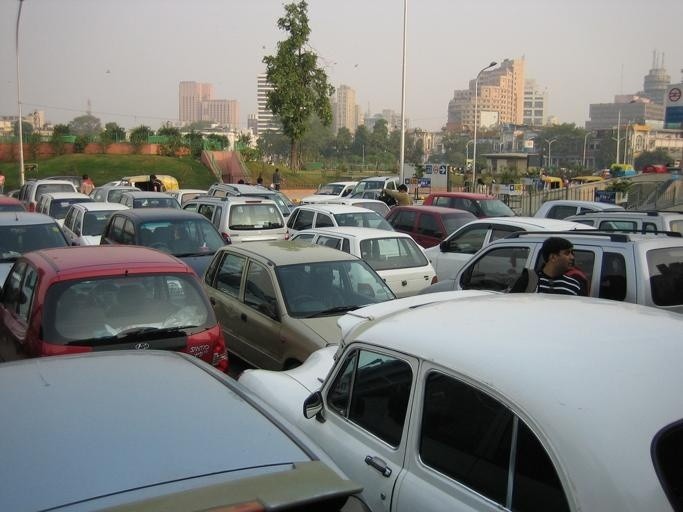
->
[664,83,682,106]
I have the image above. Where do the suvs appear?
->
[562,207,683,237]
[182,195,289,273]
[17,180,79,211]
[352,176,398,195]
[206,183,293,226]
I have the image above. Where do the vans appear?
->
[120,175,180,197]
[418,225,683,314]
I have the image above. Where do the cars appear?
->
[324,199,390,227]
[8,189,21,198]
[0,195,27,212]
[202,240,401,374]
[167,189,210,209]
[349,189,397,208]
[377,204,494,250]
[283,204,426,257]
[421,191,516,221]
[535,199,624,222]
[0,348,371,512]
[99,207,234,287]
[0,210,74,292]
[300,182,358,206]
[0,245,229,373]
[62,202,131,247]
[115,191,184,236]
[43,176,148,208]
[235,287,682,512]
[282,226,437,296]
[533,174,604,193]
[424,217,611,286]
[35,192,97,232]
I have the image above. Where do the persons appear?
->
[507,237,587,297]
[150,174,162,193]
[476,177,490,195]
[383,184,414,207]
[272,168,284,191]
[0,170,10,195]
[254,178,267,189]
[79,175,96,197]
[463,181,472,192]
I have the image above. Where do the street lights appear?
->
[465,139,473,164]
[470,58,497,185]
[542,137,557,165]
[607,94,681,163]
[582,130,592,166]
